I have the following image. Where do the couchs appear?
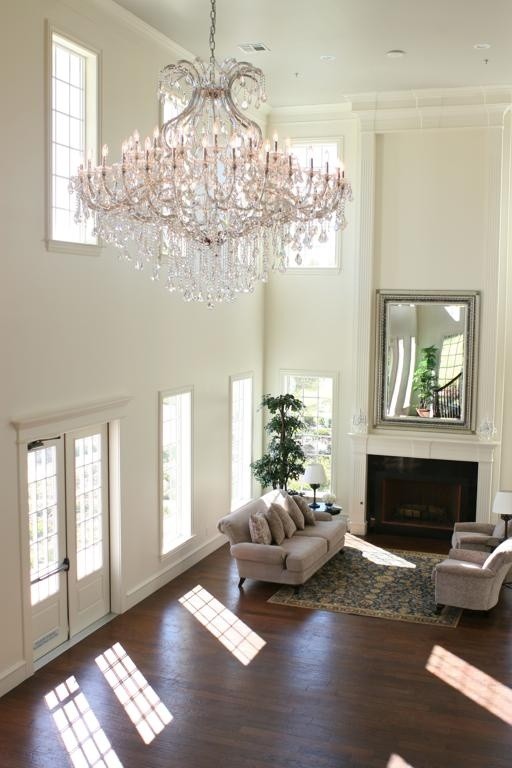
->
[217,489,346,594]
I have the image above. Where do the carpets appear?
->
[265,547,465,628]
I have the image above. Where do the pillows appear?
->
[248,494,317,545]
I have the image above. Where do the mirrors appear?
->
[372,288,481,436]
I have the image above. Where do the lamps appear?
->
[492,490,512,542]
[66,0,354,310]
[303,463,324,509]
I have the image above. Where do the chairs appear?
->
[452,516,512,554]
[431,536,512,618]
